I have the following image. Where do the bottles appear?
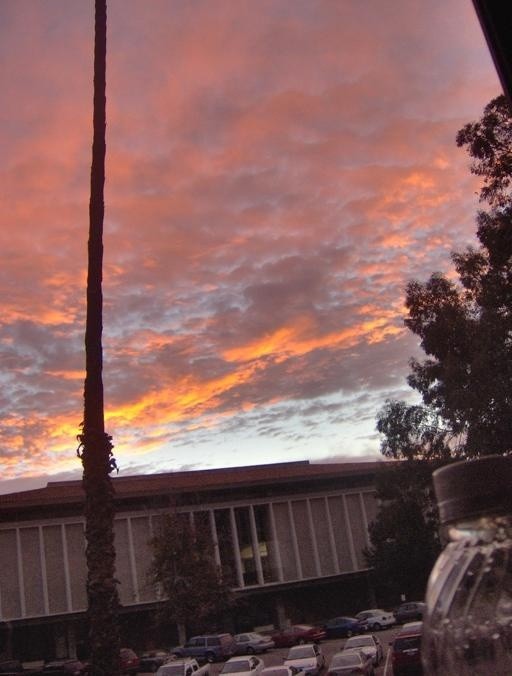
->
[419,451,512,676]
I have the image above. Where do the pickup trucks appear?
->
[153,657,211,676]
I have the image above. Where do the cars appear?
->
[43,648,178,676]
[324,617,370,638]
[271,624,328,646]
[233,632,276,654]
[218,643,328,676]
[328,647,375,676]
[341,634,383,668]
[354,609,397,632]
[394,601,426,621]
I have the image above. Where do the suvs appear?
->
[388,630,425,674]
[171,633,239,663]
